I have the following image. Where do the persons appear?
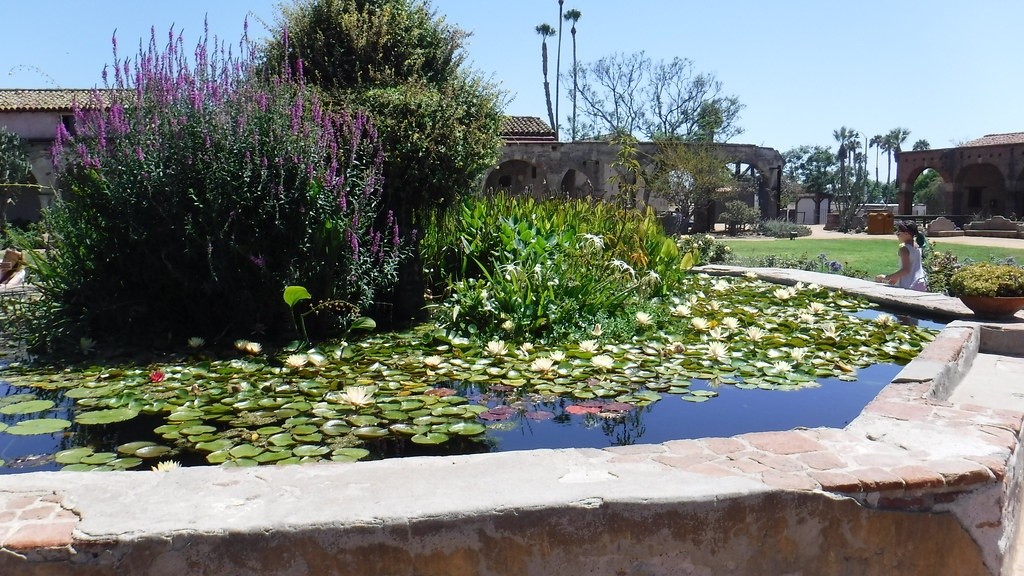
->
[884,220,927,292]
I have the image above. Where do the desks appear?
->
[655,215,677,219]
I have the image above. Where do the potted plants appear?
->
[947,261,1024,319]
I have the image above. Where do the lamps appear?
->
[790,232,798,239]
